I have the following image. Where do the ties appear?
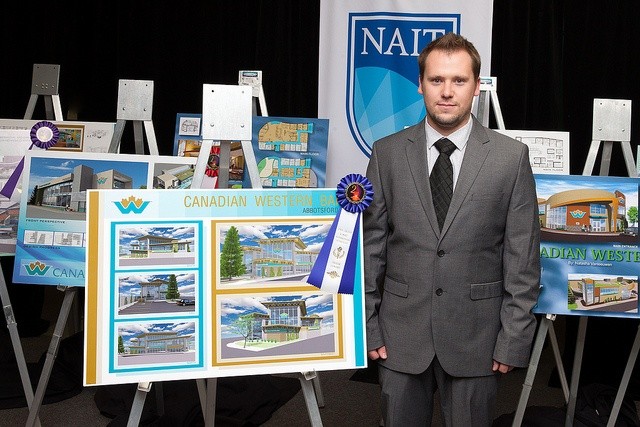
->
[428,139,457,232]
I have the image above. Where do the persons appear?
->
[362,31,541,427]
